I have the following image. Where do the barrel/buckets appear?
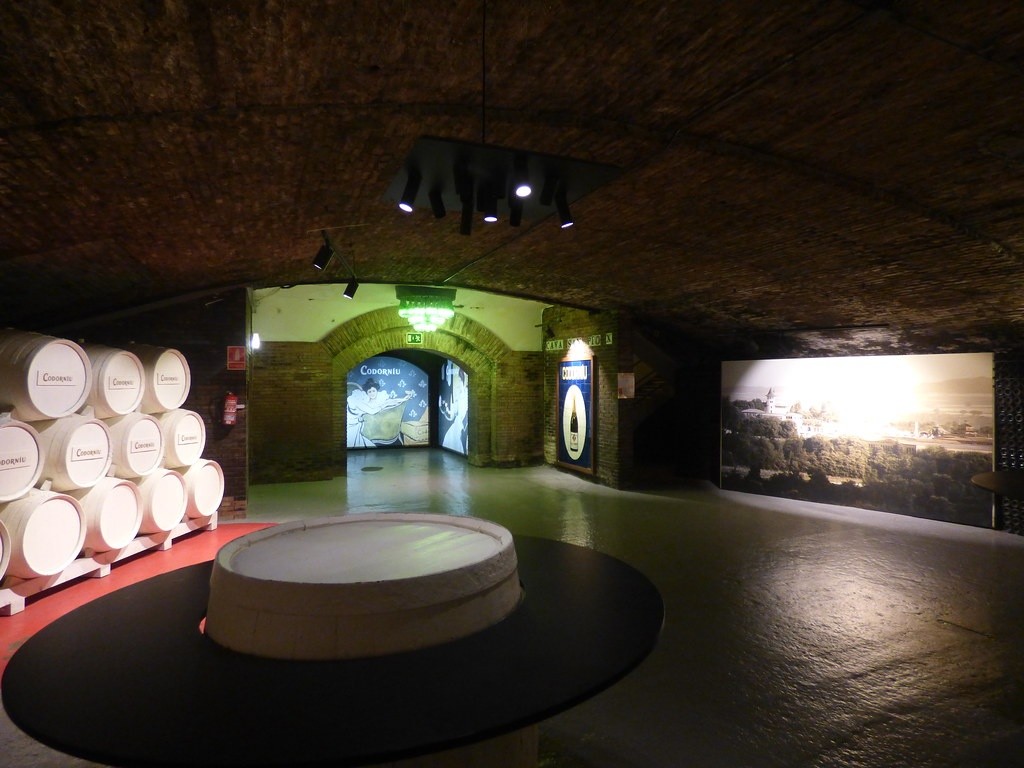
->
[1,331,224,585]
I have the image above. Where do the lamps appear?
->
[460,200,474,235]
[429,194,446,219]
[311,238,336,270]
[509,200,524,228]
[514,152,531,198]
[452,161,473,198]
[545,325,555,339]
[542,170,560,201]
[556,196,574,227]
[746,336,759,353]
[398,171,423,213]
[344,275,358,298]
[483,186,497,223]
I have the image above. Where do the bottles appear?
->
[570,396,579,452]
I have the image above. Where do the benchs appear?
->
[1,531,669,768]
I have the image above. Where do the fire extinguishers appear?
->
[222,391,238,426]
